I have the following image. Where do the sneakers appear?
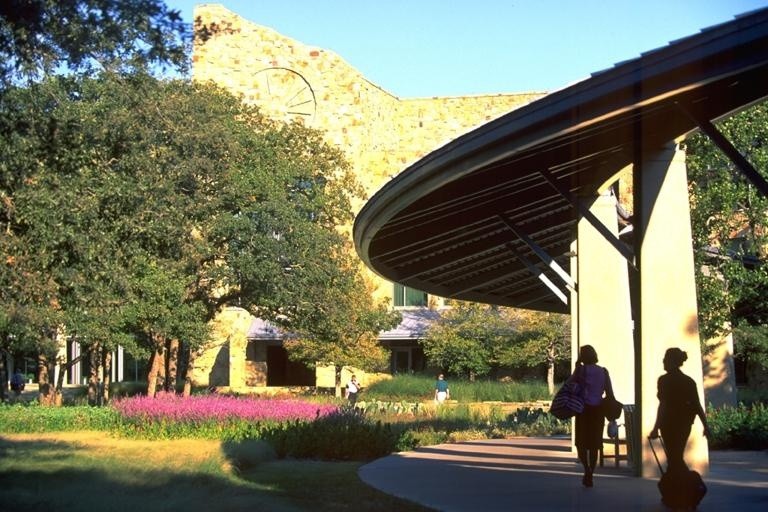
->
[582,472,594,487]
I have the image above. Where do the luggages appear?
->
[648,435,707,512]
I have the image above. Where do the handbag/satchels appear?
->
[549,382,584,421]
[600,397,623,421]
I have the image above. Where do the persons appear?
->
[549,344,624,489]
[434,373,449,401]
[11,368,24,397]
[345,373,361,407]
[649,347,710,466]
